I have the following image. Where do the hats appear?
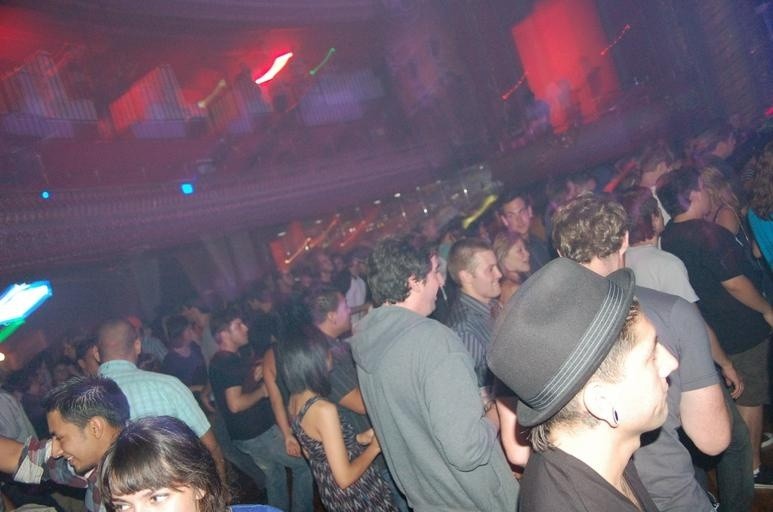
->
[484,257,636,428]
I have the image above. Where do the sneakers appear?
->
[753,463,773,490]
[760,432,773,449]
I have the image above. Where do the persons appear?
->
[0,117,772,512]
